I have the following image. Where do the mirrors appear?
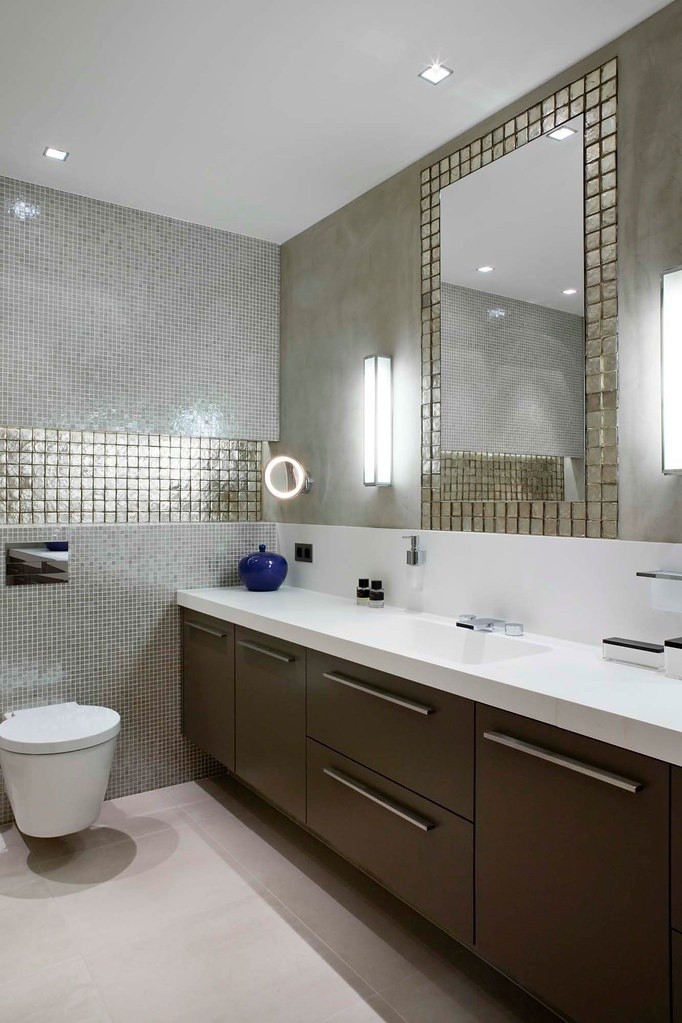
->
[440,113,586,503]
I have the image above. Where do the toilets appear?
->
[1,701,122,838]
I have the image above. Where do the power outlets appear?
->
[295,543,312,562]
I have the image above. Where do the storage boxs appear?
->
[603,637,682,680]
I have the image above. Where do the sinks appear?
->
[328,614,553,665]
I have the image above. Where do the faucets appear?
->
[456,617,507,630]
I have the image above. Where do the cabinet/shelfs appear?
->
[306,647,475,948]
[474,700,671,1023]
[182,604,233,774]
[671,764,682,1023]
[234,625,306,829]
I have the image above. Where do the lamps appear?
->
[263,453,313,501]
[363,354,393,487]
[660,265,682,475]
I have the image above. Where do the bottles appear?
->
[357,578,370,606]
[369,580,384,609]
